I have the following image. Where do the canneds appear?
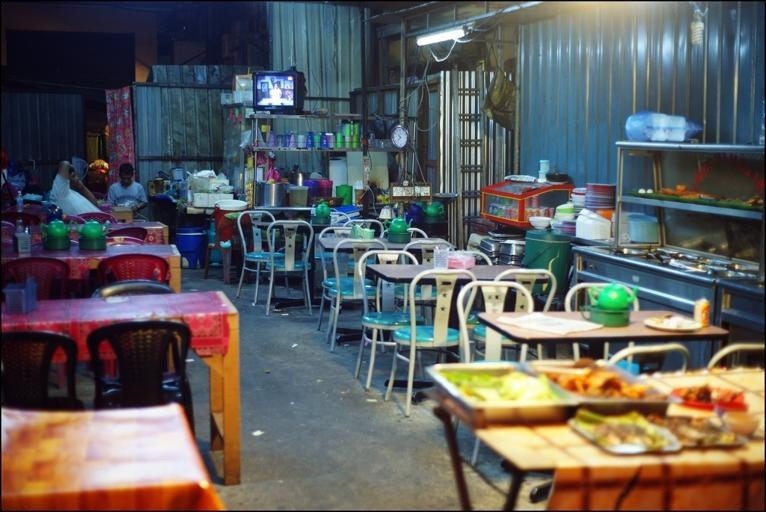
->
[487,195,554,221]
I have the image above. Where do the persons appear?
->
[106,163,148,218]
[42,159,107,219]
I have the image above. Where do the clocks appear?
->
[389,124,409,149]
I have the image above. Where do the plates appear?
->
[670,386,743,410]
[644,315,699,331]
[552,183,616,238]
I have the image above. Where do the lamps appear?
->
[688,0,707,49]
[415,24,469,47]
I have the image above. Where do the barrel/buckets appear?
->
[214,199,251,255]
[520,230,572,295]
[175,226,207,270]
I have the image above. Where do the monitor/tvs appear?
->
[253,70,305,115]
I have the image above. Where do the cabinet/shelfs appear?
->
[250,112,387,240]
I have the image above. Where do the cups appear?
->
[434,247,450,271]
[581,307,629,328]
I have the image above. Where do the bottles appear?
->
[538,159,550,182]
[16,217,24,233]
[16,191,24,212]
[268,121,361,149]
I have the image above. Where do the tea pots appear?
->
[314,201,331,217]
[73,219,109,239]
[422,203,444,217]
[39,218,75,238]
[385,214,414,233]
[585,280,638,311]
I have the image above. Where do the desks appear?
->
[1,402,226,510]
[410,367,766,510]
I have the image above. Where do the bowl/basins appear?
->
[628,215,659,243]
[528,216,551,230]
[311,215,331,226]
[546,172,567,182]
[42,237,106,250]
[387,232,411,244]
[722,412,759,436]
